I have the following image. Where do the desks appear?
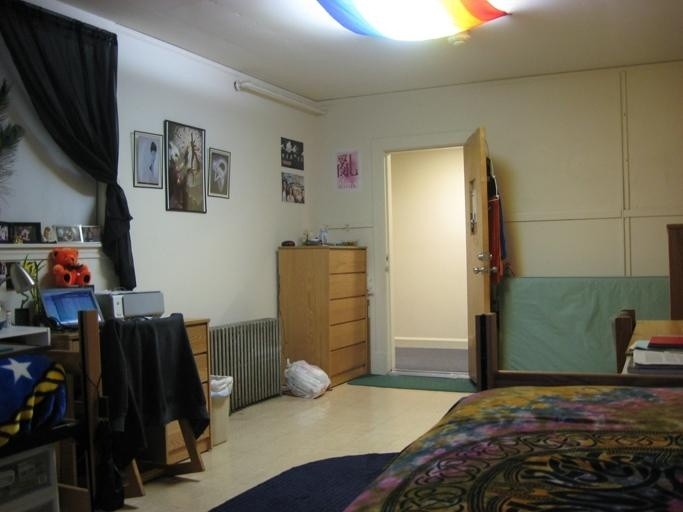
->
[624,320,683,386]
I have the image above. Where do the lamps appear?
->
[10,264,35,325]
[317,0,513,42]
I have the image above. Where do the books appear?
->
[625,335,683,376]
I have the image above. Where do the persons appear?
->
[282,180,305,204]
[145,141,158,179]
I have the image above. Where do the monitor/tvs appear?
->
[38,287,105,329]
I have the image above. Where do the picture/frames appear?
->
[281,137,305,170]
[335,151,362,190]
[281,172,306,204]
[11,222,42,243]
[1,222,11,243]
[51,224,83,243]
[134,130,163,189]
[164,119,209,214]
[81,226,102,243]
[208,147,231,199]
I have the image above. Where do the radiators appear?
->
[206,318,284,413]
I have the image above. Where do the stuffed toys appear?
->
[50,248,90,285]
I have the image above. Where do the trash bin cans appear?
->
[208,375,234,446]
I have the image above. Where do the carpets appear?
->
[210,452,397,511]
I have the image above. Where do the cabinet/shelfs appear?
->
[278,245,371,389]
[0,325,60,512]
[60,321,212,491]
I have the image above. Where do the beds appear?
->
[345,384,683,512]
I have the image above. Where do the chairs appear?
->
[614,308,636,374]
